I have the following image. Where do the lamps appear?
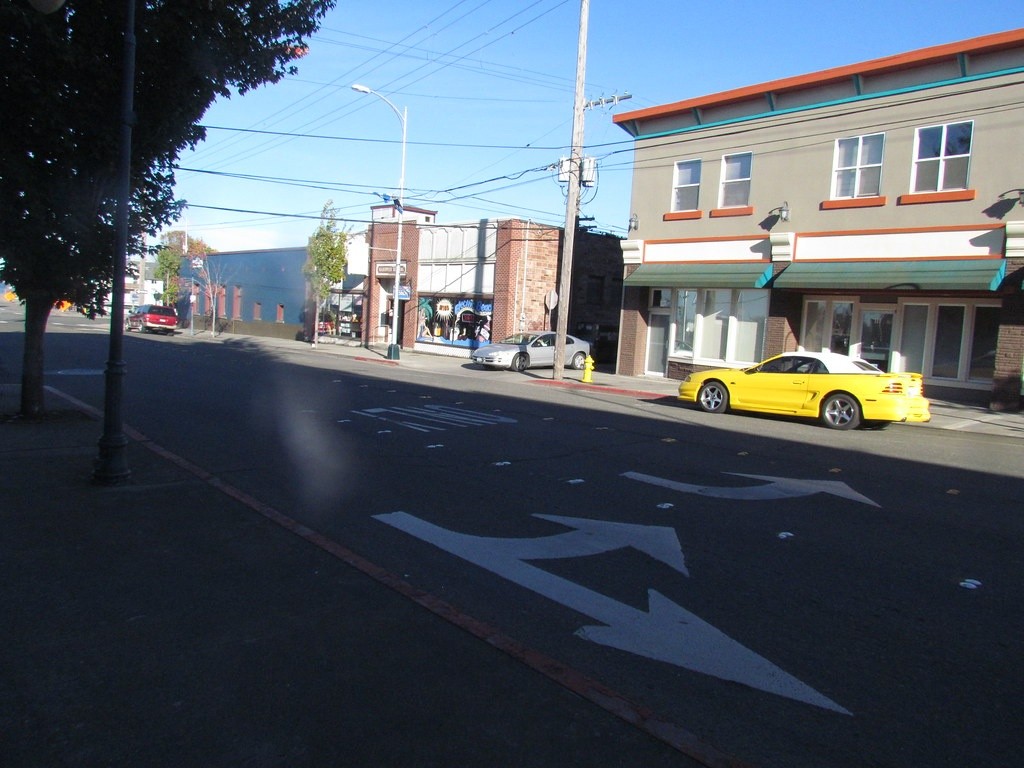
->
[1000,189,1024,205]
[630,213,639,230]
[780,203,789,220]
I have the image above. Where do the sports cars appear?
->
[678,351,933,430]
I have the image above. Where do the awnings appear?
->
[624,263,773,288]
[773,258,1006,292]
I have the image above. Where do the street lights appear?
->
[351,83,409,363]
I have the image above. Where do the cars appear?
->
[317,313,332,336]
[471,330,591,372]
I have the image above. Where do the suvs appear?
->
[125,304,179,336]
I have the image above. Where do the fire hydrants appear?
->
[580,354,597,384]
